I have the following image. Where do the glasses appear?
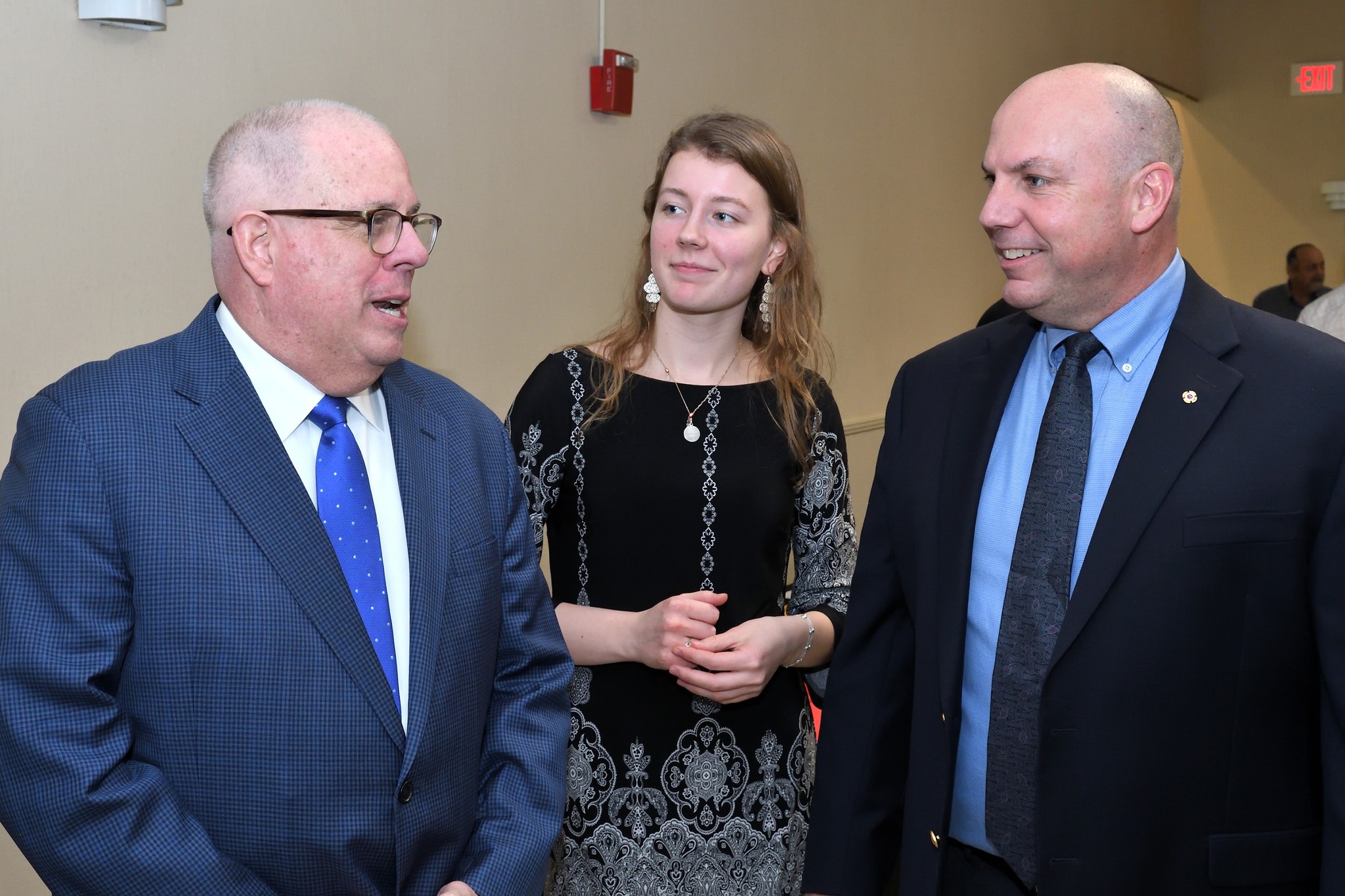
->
[226,207,442,256]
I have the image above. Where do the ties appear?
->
[983,330,1104,878]
[310,394,401,720]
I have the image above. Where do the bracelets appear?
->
[782,614,815,668]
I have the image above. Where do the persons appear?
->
[803,60,1345,896]
[505,110,857,896]
[1296,284,1345,343]
[1253,243,1334,322]
[0,99,574,896]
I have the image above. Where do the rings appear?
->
[685,637,691,647]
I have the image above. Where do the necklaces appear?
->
[653,333,743,442]
